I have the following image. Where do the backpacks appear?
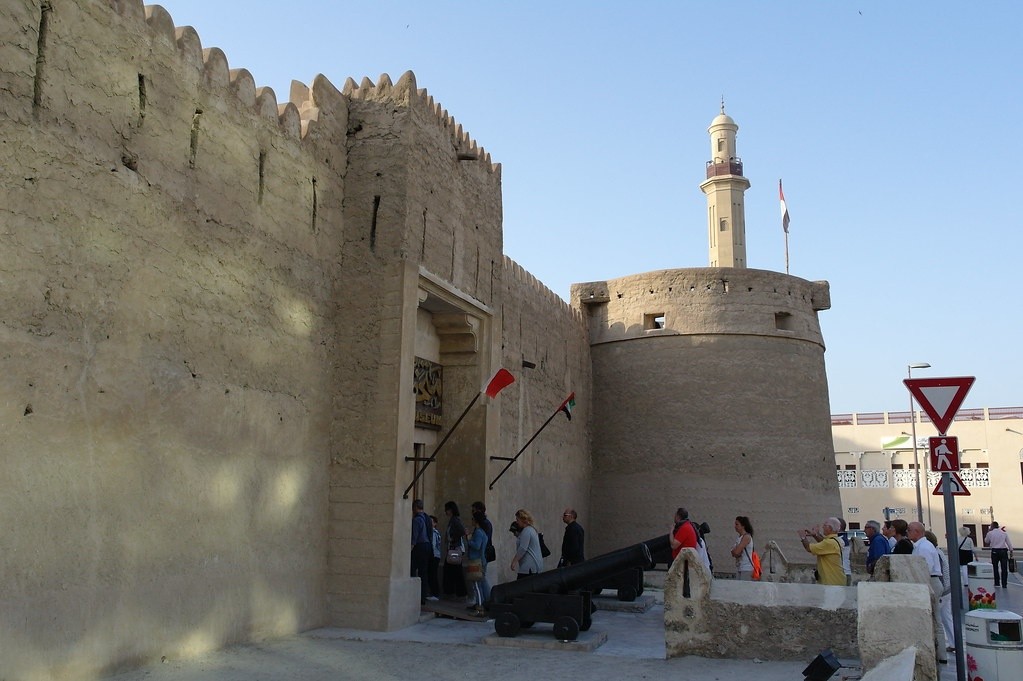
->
[741,534,762,580]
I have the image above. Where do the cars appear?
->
[846,530,870,546]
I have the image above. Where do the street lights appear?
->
[909,363,931,523]
[919,443,932,532]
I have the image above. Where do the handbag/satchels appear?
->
[466,559,483,579]
[447,549,462,564]
[1008,555,1018,573]
[557,558,571,568]
[537,532,551,558]
[484,546,496,563]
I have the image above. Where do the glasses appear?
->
[564,513,572,516]
[865,526,872,529]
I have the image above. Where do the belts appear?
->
[933,575,937,577]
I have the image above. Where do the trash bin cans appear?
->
[966,561,996,611]
[964,609,1023,681]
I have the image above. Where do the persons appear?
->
[731,516,754,580]
[670,508,712,576]
[906,522,948,664]
[411,499,442,606]
[561,509,584,567]
[882,518,913,577]
[958,527,974,586]
[444,501,468,602]
[863,520,891,577]
[925,530,957,652]
[984,521,1013,588]
[464,501,492,617]
[798,517,851,586]
[509,509,543,580]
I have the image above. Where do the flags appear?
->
[779,184,791,235]
[479,366,516,399]
[560,391,578,421]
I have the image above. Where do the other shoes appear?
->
[465,598,476,606]
[590,607,597,614]
[421,600,427,605]
[468,606,484,617]
[994,584,1000,586]
[946,647,956,652]
[427,596,439,601]
[938,659,947,664]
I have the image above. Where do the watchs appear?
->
[801,536,806,540]
